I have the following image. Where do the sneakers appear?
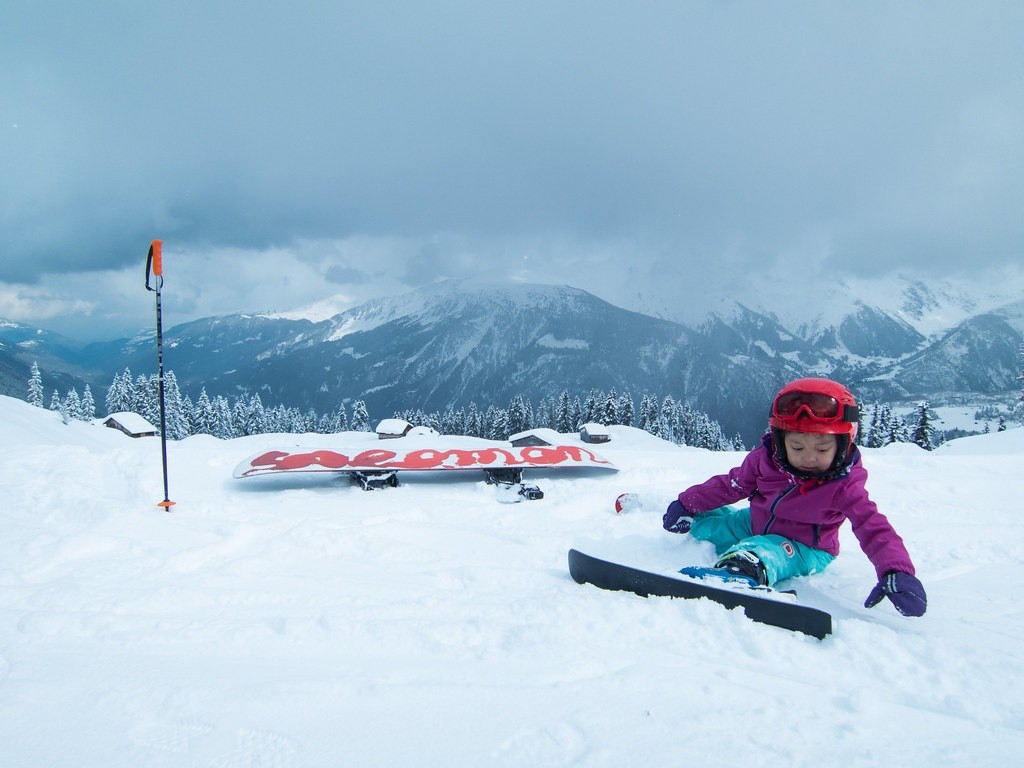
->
[679,560,760,590]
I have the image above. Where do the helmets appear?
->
[770,378,858,460]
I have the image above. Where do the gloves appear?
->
[663,499,695,534]
[865,571,927,617]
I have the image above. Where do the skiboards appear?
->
[567,491,832,638]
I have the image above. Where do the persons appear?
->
[663,377,927,618]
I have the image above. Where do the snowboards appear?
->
[231,446,620,485]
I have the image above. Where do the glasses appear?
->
[773,389,844,424]
